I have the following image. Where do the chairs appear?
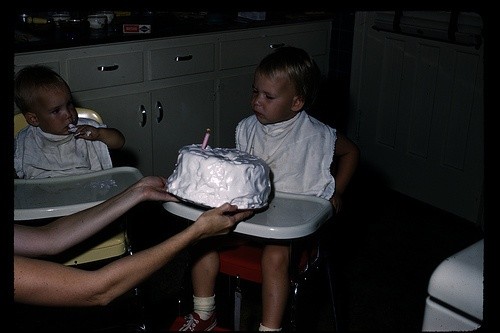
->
[14,107,148,333]
[161,190,340,333]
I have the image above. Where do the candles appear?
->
[202,128,211,149]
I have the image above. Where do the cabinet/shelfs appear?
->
[216,31,327,148]
[68,42,214,178]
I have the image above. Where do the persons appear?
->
[14,176,256,309]
[177,49,351,332]
[14,64,126,201]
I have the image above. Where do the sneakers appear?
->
[177,310,217,333]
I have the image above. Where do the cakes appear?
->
[165,143,271,209]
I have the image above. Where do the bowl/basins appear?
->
[87,15,107,29]
[53,11,70,23]
[95,10,114,24]
